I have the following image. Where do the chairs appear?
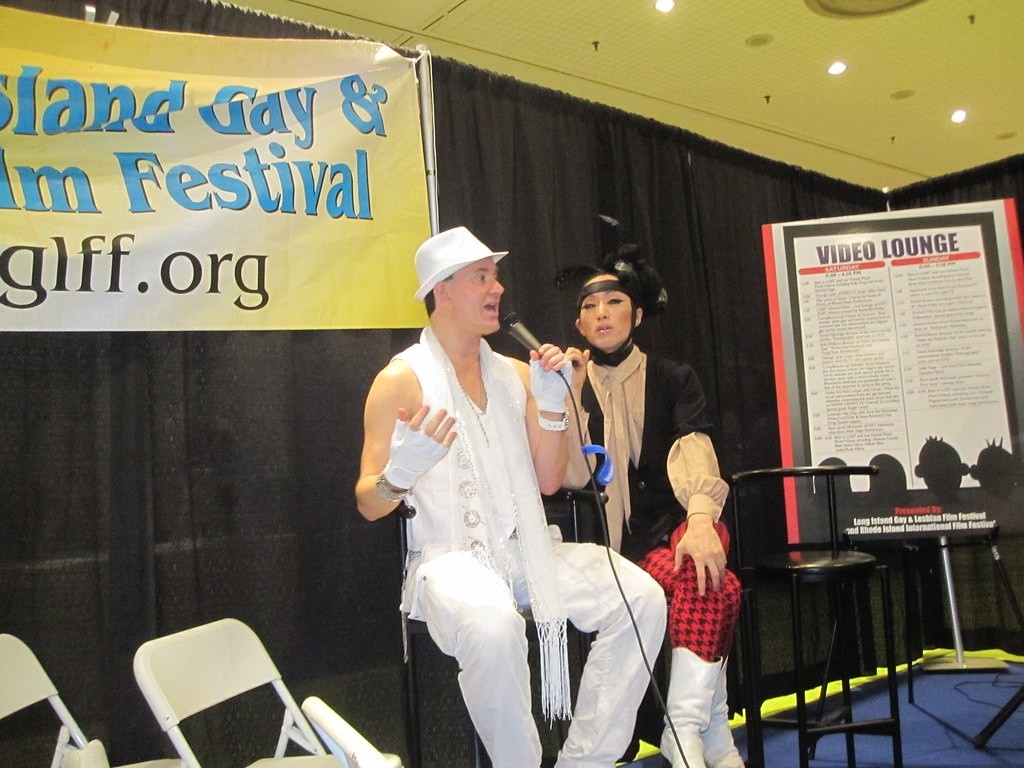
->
[396,480,609,767]
[1,618,402,767]
[728,463,903,767]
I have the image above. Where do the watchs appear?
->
[537,412,571,432]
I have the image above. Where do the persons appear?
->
[536,240,748,768]
[355,226,668,768]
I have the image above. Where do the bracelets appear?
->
[374,475,410,503]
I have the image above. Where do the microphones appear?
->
[500,312,563,377]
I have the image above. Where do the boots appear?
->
[660,647,746,768]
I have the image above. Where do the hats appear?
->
[413,225,509,303]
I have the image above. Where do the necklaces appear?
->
[451,351,489,446]
